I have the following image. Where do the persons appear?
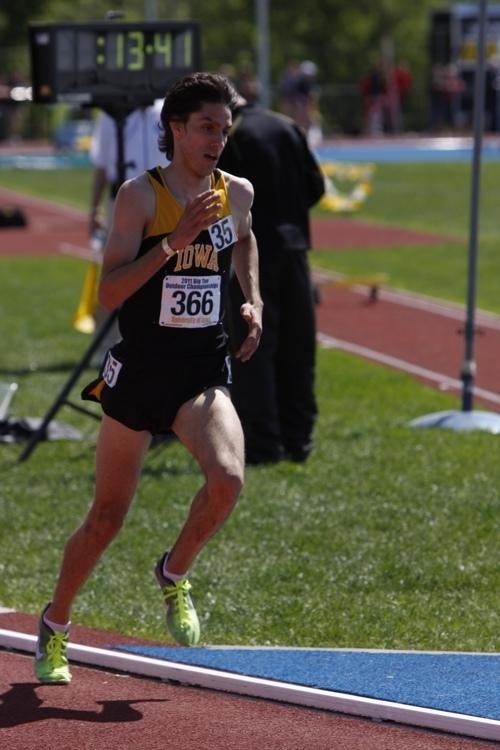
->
[213,67,328,470]
[32,71,266,683]
[80,88,175,363]
[236,48,499,151]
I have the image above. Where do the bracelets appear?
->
[160,236,177,264]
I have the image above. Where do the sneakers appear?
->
[34,603,72,685]
[155,552,201,647]
